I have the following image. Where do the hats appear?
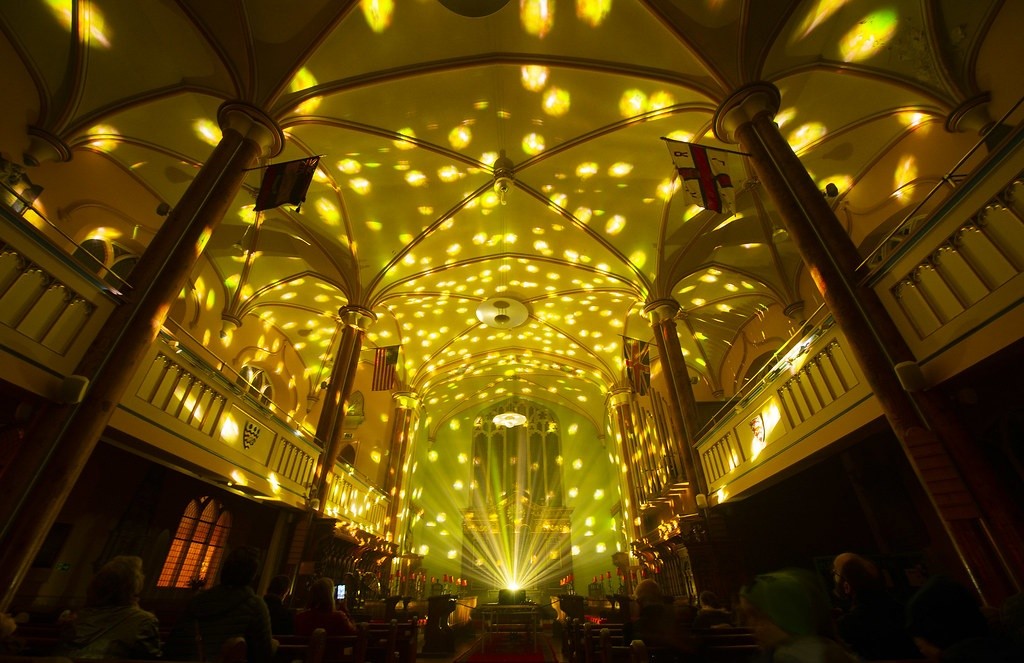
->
[740,566,813,636]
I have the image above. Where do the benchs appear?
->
[561,614,1024,663]
[0,615,418,663]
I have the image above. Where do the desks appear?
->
[480,603,540,654]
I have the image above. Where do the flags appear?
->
[371,345,399,391]
[664,140,738,216]
[254,156,321,213]
[623,336,650,396]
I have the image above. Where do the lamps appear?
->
[493,411,527,428]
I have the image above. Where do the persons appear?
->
[56,547,358,663]
[624,576,1024,663]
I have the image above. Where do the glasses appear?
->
[831,569,852,588]
